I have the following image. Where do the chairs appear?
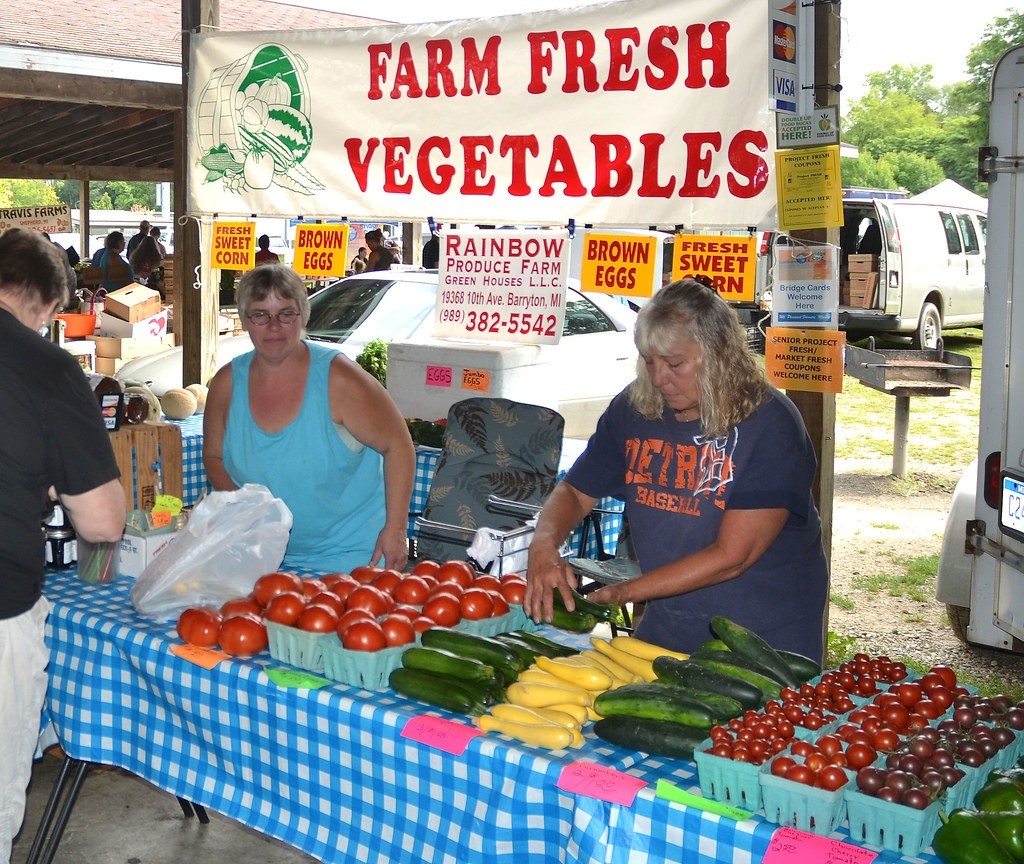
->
[575,501,650,636]
[416,397,564,565]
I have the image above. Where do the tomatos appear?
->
[253,558,528,652]
[176,597,268,654]
[704,654,1024,812]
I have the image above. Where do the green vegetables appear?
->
[352,338,445,447]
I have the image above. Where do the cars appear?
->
[111,263,648,443]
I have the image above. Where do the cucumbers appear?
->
[595,616,821,760]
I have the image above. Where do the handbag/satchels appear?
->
[130,484,293,617]
[147,266,166,301]
[65,246,80,268]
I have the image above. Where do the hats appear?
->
[139,221,153,230]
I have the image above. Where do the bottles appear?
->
[123,392,149,427]
[39,521,77,570]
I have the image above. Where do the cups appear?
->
[76,535,118,583]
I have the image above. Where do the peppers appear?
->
[932,757,1024,864]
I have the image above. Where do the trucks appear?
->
[932,38,1023,662]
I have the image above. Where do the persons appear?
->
[349,226,401,275]
[0,226,126,864]
[203,262,416,572]
[45,218,167,295]
[421,230,440,270]
[522,280,830,674]
[255,233,280,265]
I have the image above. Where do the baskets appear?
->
[263,597,546,691]
[691,654,1024,857]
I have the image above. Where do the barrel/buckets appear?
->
[55,309,96,338]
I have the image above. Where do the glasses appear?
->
[42,293,64,321]
[244,307,300,325]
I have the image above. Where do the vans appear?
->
[752,178,988,355]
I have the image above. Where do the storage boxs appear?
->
[86,283,169,374]
[384,337,542,422]
[696,655,1023,856]
[267,604,542,688]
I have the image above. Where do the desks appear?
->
[27,560,1022,864]
